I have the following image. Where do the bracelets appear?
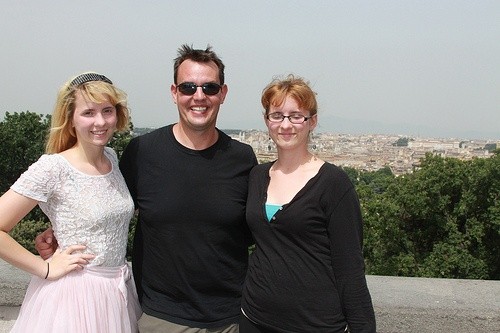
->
[45,260,50,279]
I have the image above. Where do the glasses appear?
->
[267,111,311,124]
[176,82,222,95]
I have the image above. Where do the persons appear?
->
[0,71,143,333]
[34,42,259,333]
[239,73,376,333]
